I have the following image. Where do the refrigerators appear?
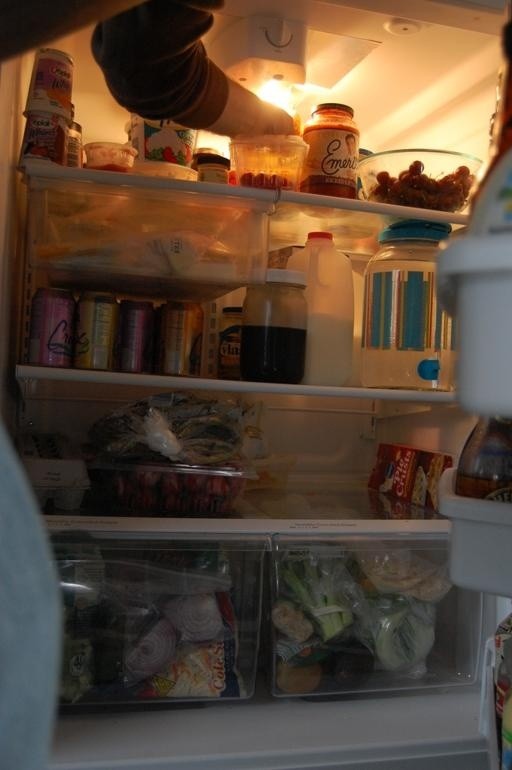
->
[2,0,512,769]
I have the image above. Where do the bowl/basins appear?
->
[82,141,139,172]
[227,142,309,190]
[359,147,485,215]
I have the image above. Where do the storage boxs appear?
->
[263,532,483,699]
[28,174,275,296]
[51,536,266,710]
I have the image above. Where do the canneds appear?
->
[219,306,243,380]
[300,102,360,199]
[161,300,203,378]
[240,267,307,384]
[76,293,121,370]
[119,302,157,373]
[28,285,75,369]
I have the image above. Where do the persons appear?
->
[0,1,302,158]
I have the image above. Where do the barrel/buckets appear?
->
[284,230,358,389]
